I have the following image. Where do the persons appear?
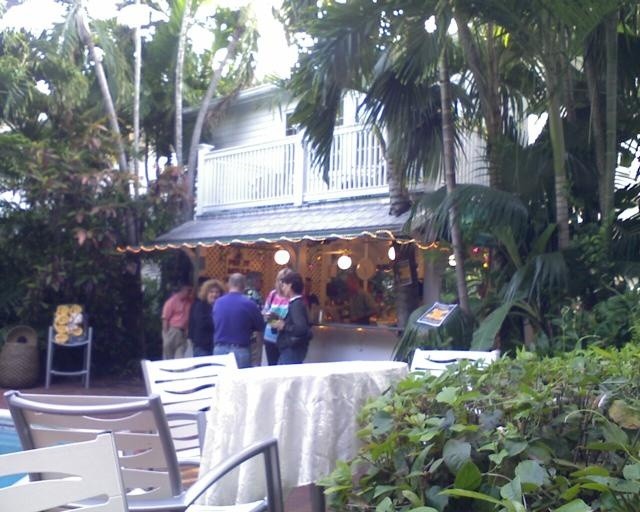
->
[190,278,227,411]
[277,271,314,364]
[160,282,194,360]
[260,267,293,365]
[211,272,264,369]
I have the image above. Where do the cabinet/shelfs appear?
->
[44,327,93,388]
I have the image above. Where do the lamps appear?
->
[274,244,290,265]
[337,249,352,270]
[387,247,396,261]
[448,254,457,266]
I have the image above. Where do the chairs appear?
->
[1,388,183,508]
[410,348,501,376]
[140,350,239,491]
[1,429,285,510]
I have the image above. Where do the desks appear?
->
[195,360,412,510]
